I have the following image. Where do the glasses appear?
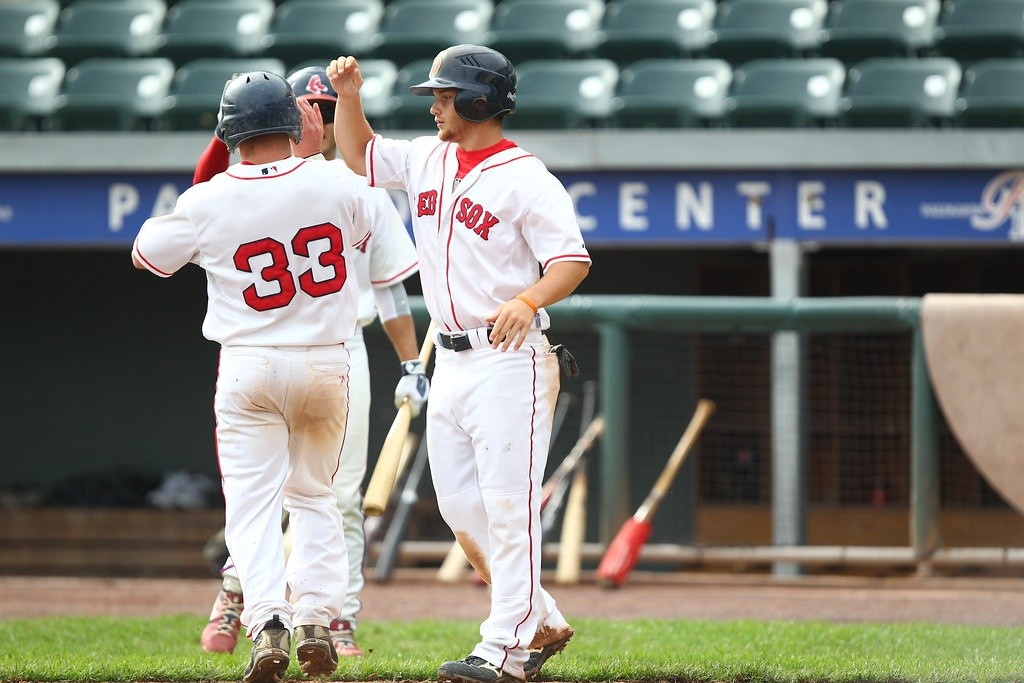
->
[315,105,335,125]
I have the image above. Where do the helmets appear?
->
[220,71,303,153]
[287,67,338,101]
[410,44,517,121]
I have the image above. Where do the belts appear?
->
[437,313,540,352]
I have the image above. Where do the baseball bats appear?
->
[361,318,436,515]
[285,415,611,584]
[555,381,594,585]
[595,398,717,587]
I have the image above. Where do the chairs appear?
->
[0,0,1024,135]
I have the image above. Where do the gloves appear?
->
[214,73,245,142]
[395,359,431,419]
[547,343,580,378]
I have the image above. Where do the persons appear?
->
[130,65,428,683]
[327,45,592,683]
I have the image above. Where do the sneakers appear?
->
[329,620,365,656]
[523,632,573,681]
[244,614,291,683]
[439,656,524,683]
[296,625,338,677]
[200,589,245,655]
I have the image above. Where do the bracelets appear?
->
[516,294,538,317]
